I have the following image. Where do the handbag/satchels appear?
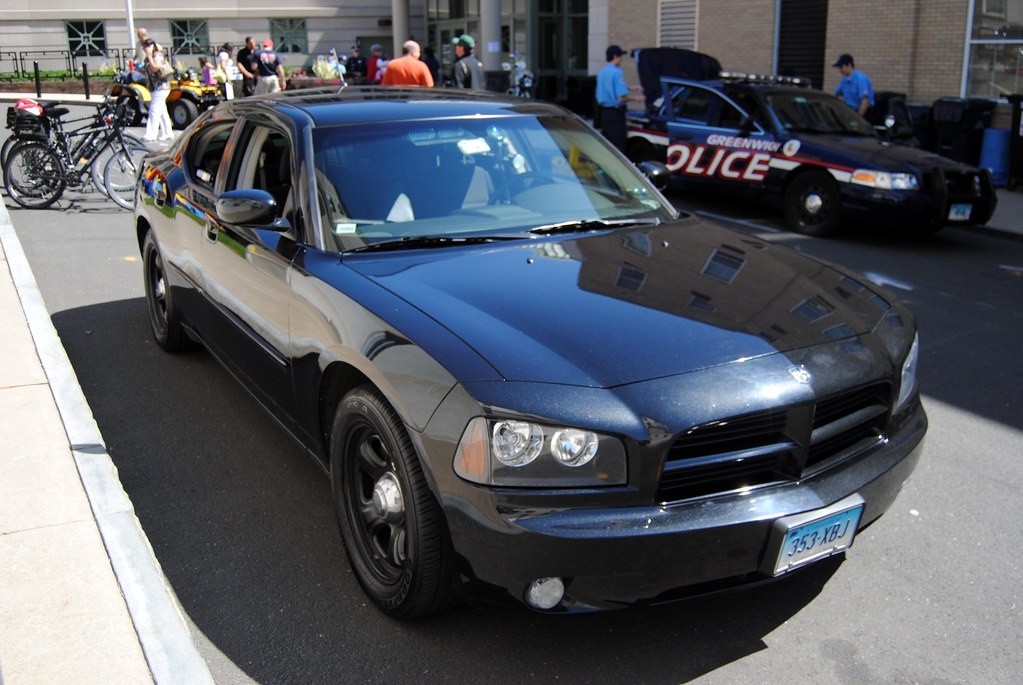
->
[213,64,226,83]
[157,61,175,80]
[225,82,235,100]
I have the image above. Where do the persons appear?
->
[337,54,350,78]
[382,40,434,88]
[237,36,258,96]
[215,42,234,100]
[367,43,390,82]
[134,28,162,63]
[141,39,173,141]
[347,46,367,78]
[833,54,876,125]
[421,47,441,80]
[451,35,485,88]
[199,57,218,87]
[251,38,287,95]
[594,45,644,150]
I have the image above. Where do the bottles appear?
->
[81,137,101,160]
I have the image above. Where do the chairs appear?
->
[424,138,506,213]
[279,141,328,214]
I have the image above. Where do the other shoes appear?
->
[159,134,174,141]
[141,135,157,140]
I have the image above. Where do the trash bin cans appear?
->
[485,70,512,93]
[866,90,929,141]
[936,95,997,167]
[1007,92,1023,196]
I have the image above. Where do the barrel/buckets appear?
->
[979,128,1010,187]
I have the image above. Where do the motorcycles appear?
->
[114,68,222,128]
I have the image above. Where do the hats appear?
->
[263,39,274,47]
[351,46,361,50]
[338,54,347,61]
[606,45,627,62]
[370,44,382,53]
[451,34,475,49]
[832,54,853,67]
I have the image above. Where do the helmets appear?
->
[14,99,44,117]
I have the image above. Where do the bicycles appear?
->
[2,75,157,212]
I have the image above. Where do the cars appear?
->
[135,85,928,623]
[625,46,999,244]
